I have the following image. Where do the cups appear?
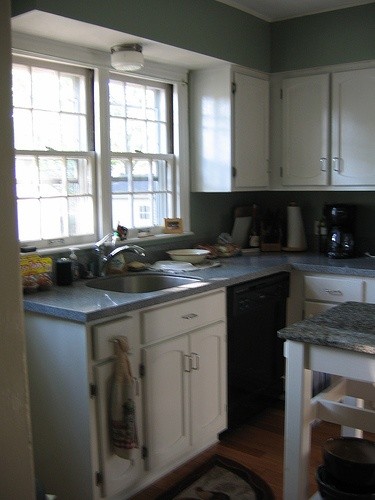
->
[54,260,72,287]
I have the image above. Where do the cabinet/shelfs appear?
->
[280,68,375,187]
[189,65,270,192]
[23,286,227,500]
[303,275,364,319]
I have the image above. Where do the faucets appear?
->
[101,243,149,271]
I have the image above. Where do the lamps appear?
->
[110,44,144,71]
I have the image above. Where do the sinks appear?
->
[77,271,207,299]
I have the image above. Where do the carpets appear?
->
[154,454,274,500]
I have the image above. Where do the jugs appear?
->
[318,202,360,259]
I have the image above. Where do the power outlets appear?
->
[314,221,327,235]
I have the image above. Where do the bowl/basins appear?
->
[315,464,375,500]
[322,437,375,479]
[165,248,210,263]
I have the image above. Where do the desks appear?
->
[278,301,375,500]
